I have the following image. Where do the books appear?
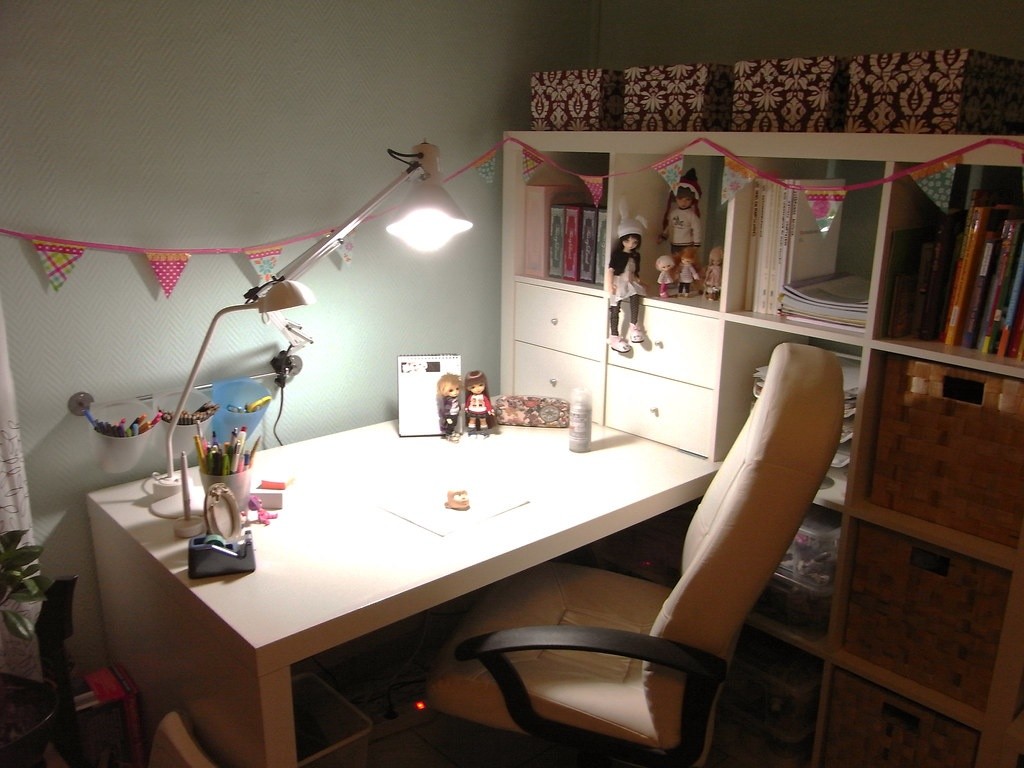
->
[524,185,607,287]
[880,188,1024,363]
[74,661,146,768]
[751,364,858,470]
[742,176,868,338]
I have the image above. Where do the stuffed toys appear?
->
[436,372,463,442]
[464,371,495,439]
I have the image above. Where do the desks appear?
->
[88,396,719,768]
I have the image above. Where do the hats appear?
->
[662,167,702,231]
[617,196,648,238]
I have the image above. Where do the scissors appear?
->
[247,393,272,413]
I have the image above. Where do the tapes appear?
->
[204,532,252,548]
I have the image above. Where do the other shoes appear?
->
[659,280,721,298]
[606,334,632,353]
[629,322,645,343]
[446,427,490,443]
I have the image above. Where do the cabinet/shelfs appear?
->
[500,129,1024,768]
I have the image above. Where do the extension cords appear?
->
[370,701,436,743]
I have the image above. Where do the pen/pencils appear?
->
[81,408,161,438]
[159,399,221,426]
[181,451,190,521]
[193,418,263,476]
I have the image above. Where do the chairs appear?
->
[420,342,844,768]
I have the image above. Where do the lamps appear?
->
[149,141,477,520]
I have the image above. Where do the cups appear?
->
[213,378,272,445]
[154,391,215,458]
[90,397,154,474]
[197,465,251,514]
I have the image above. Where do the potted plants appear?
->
[0,530,60,768]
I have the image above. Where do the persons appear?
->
[656,167,703,299]
[605,202,645,352]
[705,245,724,301]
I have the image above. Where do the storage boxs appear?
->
[843,519,1013,712]
[724,630,823,736]
[778,504,841,586]
[823,670,980,768]
[716,698,816,768]
[529,69,623,131]
[622,62,734,132]
[846,47,1024,135]
[870,354,1024,551]
[294,672,375,768]
[730,55,849,134]
[752,567,833,642]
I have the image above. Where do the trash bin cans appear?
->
[289,669,374,768]
